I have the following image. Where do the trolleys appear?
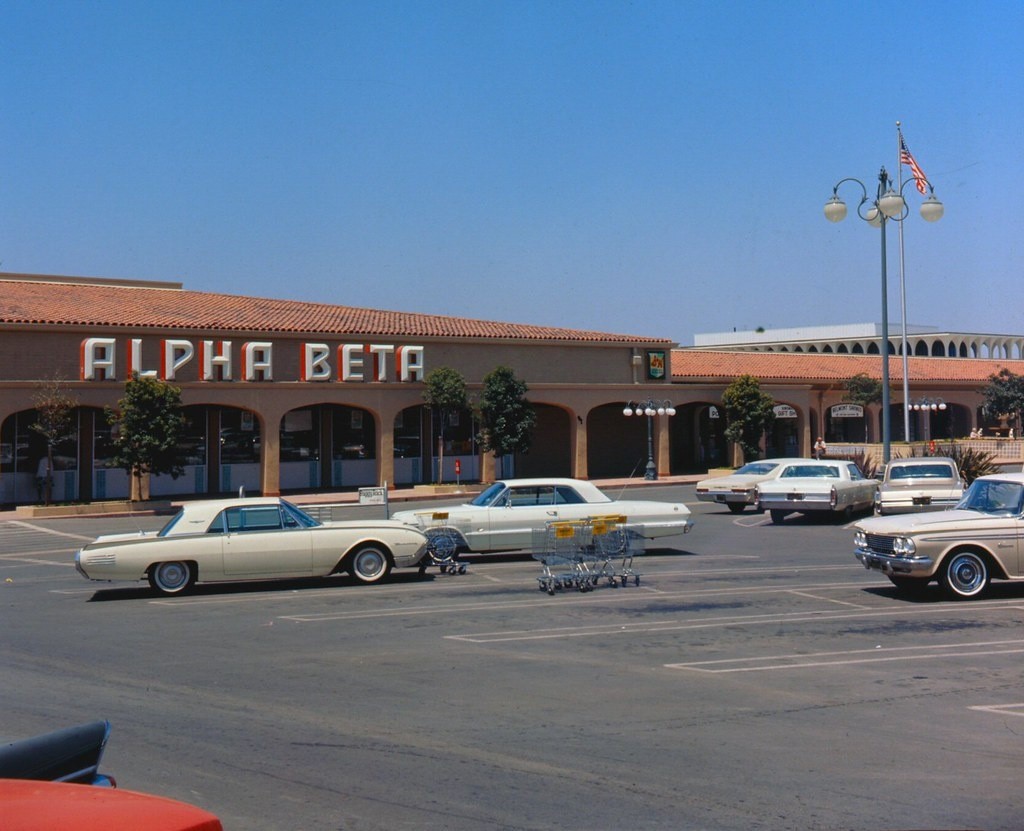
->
[532,513,647,597]
[409,511,474,578]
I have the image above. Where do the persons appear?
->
[814,437,826,459]
[978,428,983,437]
[1009,428,1013,438]
[970,428,979,438]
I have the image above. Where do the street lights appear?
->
[907,394,947,451]
[623,395,676,481]
[821,166,945,470]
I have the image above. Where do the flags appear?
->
[901,132,927,194]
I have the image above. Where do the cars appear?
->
[0,442,78,470]
[251,433,300,461]
[754,459,881,524]
[874,457,968,516]
[393,435,420,456]
[389,477,695,562]
[851,471,1024,600]
[695,460,781,513]
[75,495,431,595]
[337,441,371,459]
[193,427,249,463]
